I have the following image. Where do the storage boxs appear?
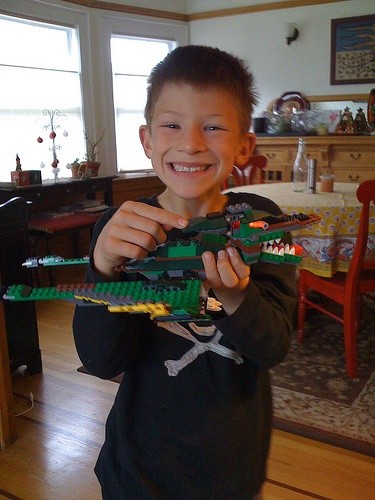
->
[11,170,41,186]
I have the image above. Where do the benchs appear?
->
[28,205,106,288]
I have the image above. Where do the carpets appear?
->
[78,287,374,458]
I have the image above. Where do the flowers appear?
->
[67,159,79,169]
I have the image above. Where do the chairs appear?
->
[223,156,267,189]
[296,180,375,379]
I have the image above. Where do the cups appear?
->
[320,175,334,192]
[253,118,268,134]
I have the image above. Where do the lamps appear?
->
[283,21,299,45]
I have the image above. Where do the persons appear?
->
[73,44,297,500]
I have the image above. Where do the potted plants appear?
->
[80,128,105,177]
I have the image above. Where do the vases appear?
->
[71,164,80,177]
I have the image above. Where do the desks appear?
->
[0,175,119,259]
[220,183,375,278]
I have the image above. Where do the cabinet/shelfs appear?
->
[254,136,375,183]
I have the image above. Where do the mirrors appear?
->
[268,94,369,122]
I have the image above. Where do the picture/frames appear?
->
[329,15,375,85]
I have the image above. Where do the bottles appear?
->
[291,137,309,191]
